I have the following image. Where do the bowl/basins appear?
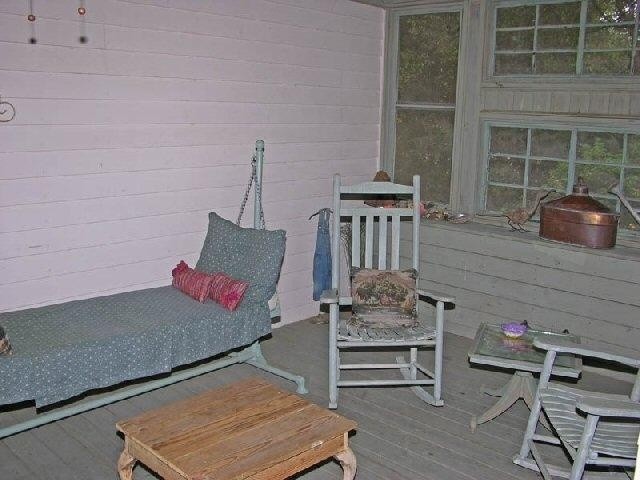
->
[501,322,526,338]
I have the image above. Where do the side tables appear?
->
[466,319,582,433]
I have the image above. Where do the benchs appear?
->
[0,135,311,439]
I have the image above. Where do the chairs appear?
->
[317,171,455,408]
[513,333,640,480]
